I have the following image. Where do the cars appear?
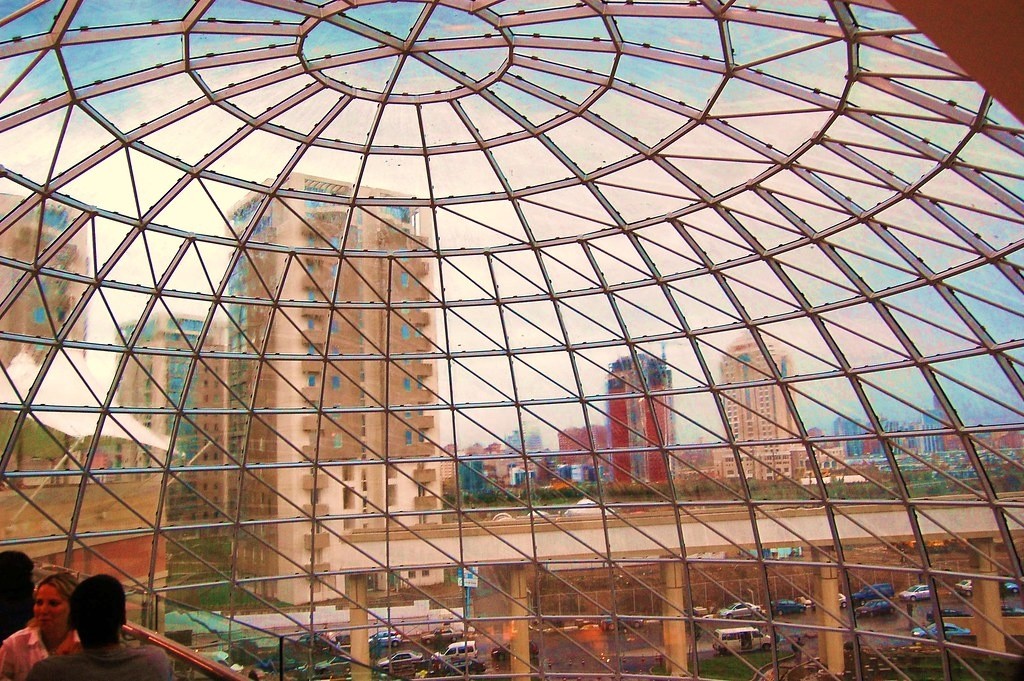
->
[926,608,972,623]
[600,615,645,631]
[428,658,486,677]
[955,580,973,598]
[910,622,971,642]
[811,593,847,610]
[898,583,931,602]
[368,631,403,648]
[716,601,763,620]
[296,634,321,644]
[1003,582,1018,593]
[314,654,351,676]
[771,598,806,617]
[1001,605,1024,617]
[854,599,896,618]
[491,637,539,661]
[420,626,463,646]
[249,654,309,674]
[377,650,424,671]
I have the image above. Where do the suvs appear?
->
[851,582,895,607]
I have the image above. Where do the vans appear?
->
[430,640,478,664]
[711,626,771,656]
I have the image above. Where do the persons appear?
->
[0,570,79,681]
[0,550,41,644]
[906,601,913,617]
[23,573,175,681]
